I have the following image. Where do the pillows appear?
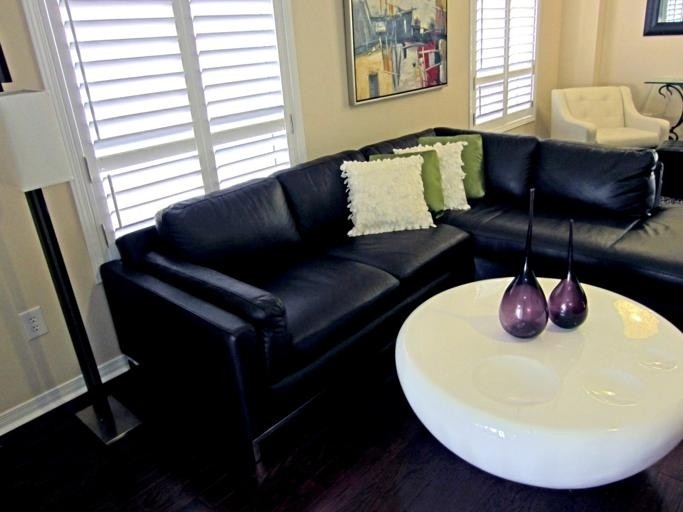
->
[392,141,471,212]
[340,154,437,237]
[370,151,444,213]
[418,133,485,199]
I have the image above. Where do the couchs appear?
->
[101,128,682,482]
[551,85,669,151]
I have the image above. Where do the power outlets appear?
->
[18,306,48,343]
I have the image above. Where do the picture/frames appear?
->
[344,0,448,107]
[643,0,683,36]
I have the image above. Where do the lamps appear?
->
[1,89,142,447]
